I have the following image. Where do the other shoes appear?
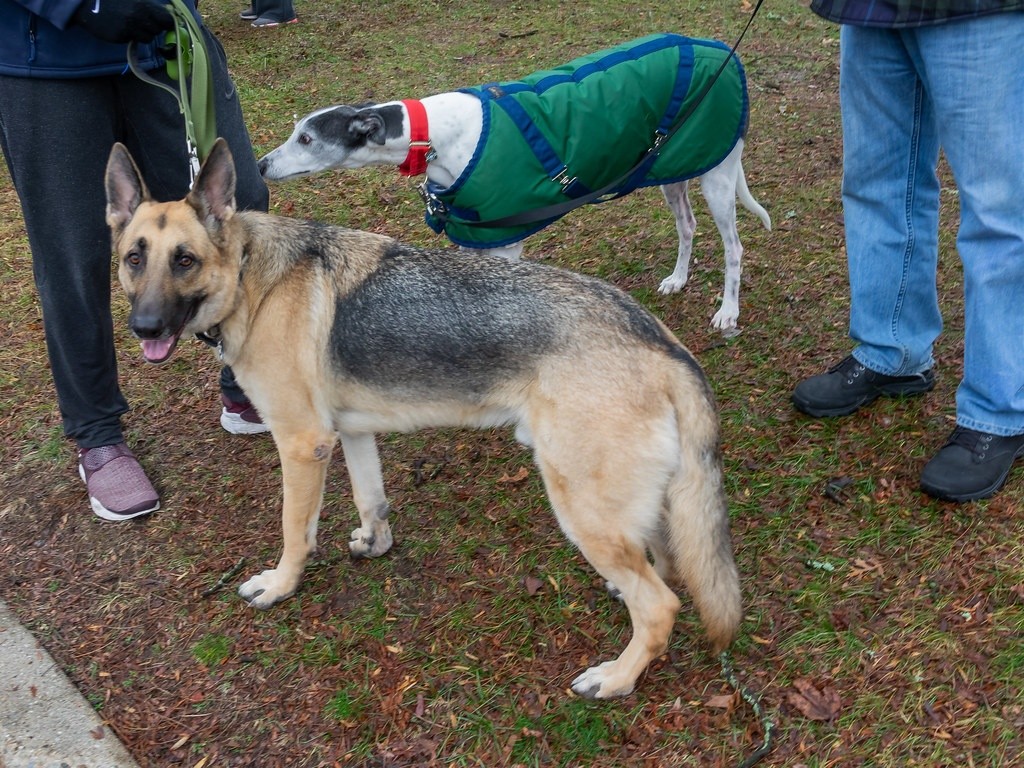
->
[239,8,258,19]
[251,17,298,28]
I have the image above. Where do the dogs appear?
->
[104,137,742,698]
[258,33,771,330]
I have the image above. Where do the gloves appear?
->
[72,0,174,43]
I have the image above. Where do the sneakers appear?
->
[220,388,271,434]
[79,442,161,520]
[793,354,935,418]
[919,425,1024,504]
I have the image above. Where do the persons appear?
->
[1,0,273,522]
[792,3,1024,500]
[239,0,298,27]
